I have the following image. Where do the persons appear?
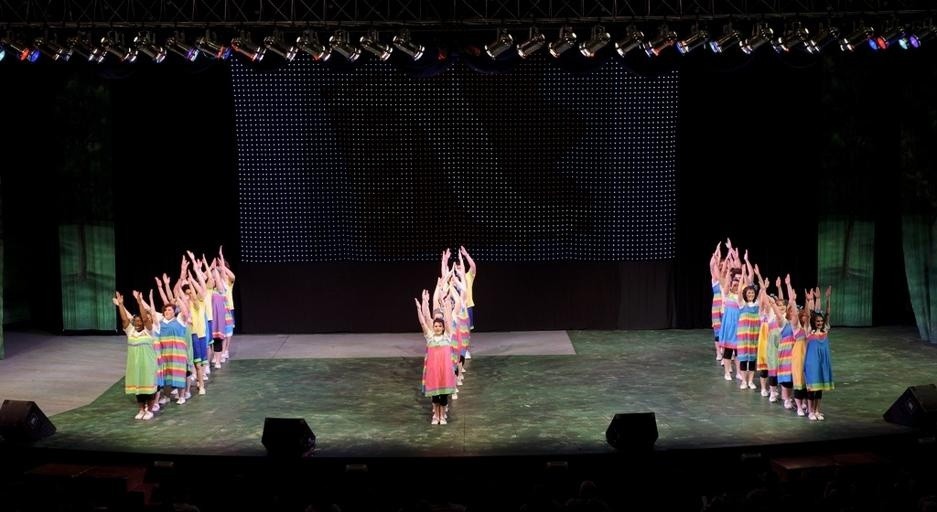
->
[709,237,835,422]
[112,245,236,421]
[1,471,937,510]
[414,245,477,425]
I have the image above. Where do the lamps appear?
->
[0,18,436,72]
[469,7,936,57]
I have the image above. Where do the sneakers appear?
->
[451,367,466,400]
[170,389,192,404]
[716,345,734,381]
[202,364,211,380]
[761,389,794,409]
[431,404,449,425]
[135,403,161,420]
[198,387,206,395]
[797,402,824,421]
[212,350,229,369]
[159,394,171,405]
[465,349,471,360]
[735,375,757,390]
